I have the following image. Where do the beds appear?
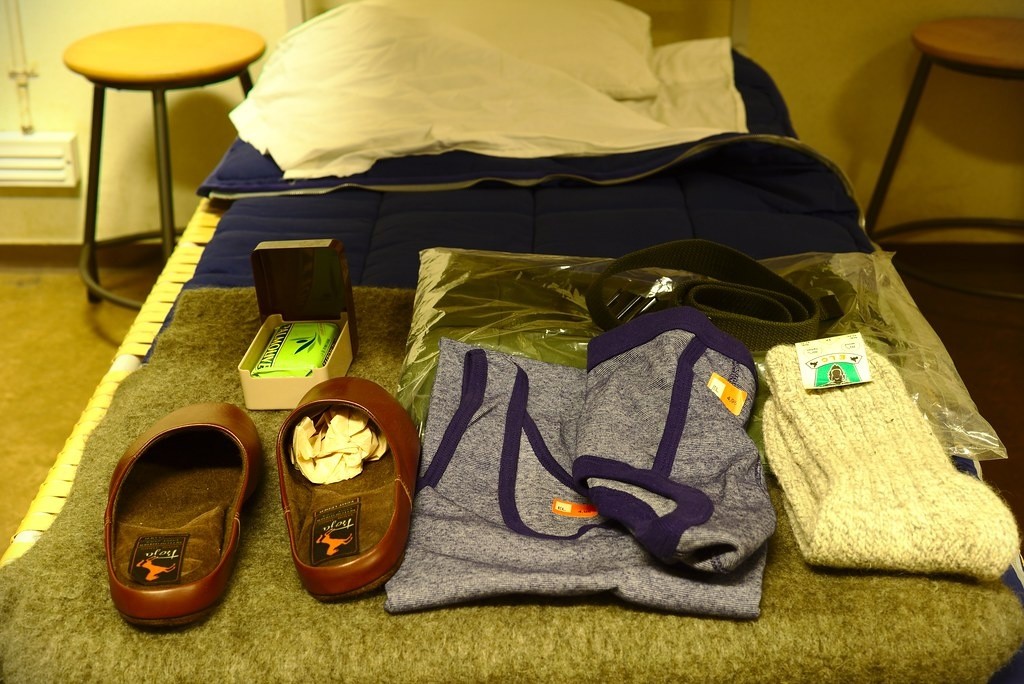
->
[2,1,1024,684]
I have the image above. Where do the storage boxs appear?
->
[235,312,352,411]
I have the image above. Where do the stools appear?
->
[64,21,268,311]
[865,16,1023,303]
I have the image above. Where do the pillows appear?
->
[246,0,664,104]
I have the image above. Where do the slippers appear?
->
[275,374,421,602]
[104,401,264,627]
